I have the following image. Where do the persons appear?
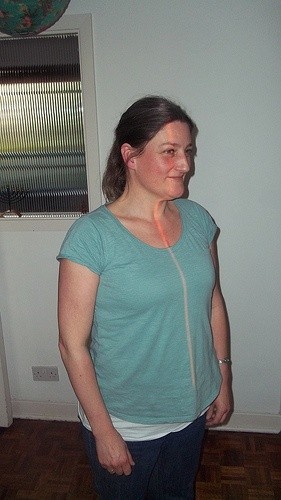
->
[56,97,234,500]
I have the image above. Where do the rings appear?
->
[111,469,115,473]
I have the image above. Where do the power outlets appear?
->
[32,366,59,381]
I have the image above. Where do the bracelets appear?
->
[217,357,231,364]
